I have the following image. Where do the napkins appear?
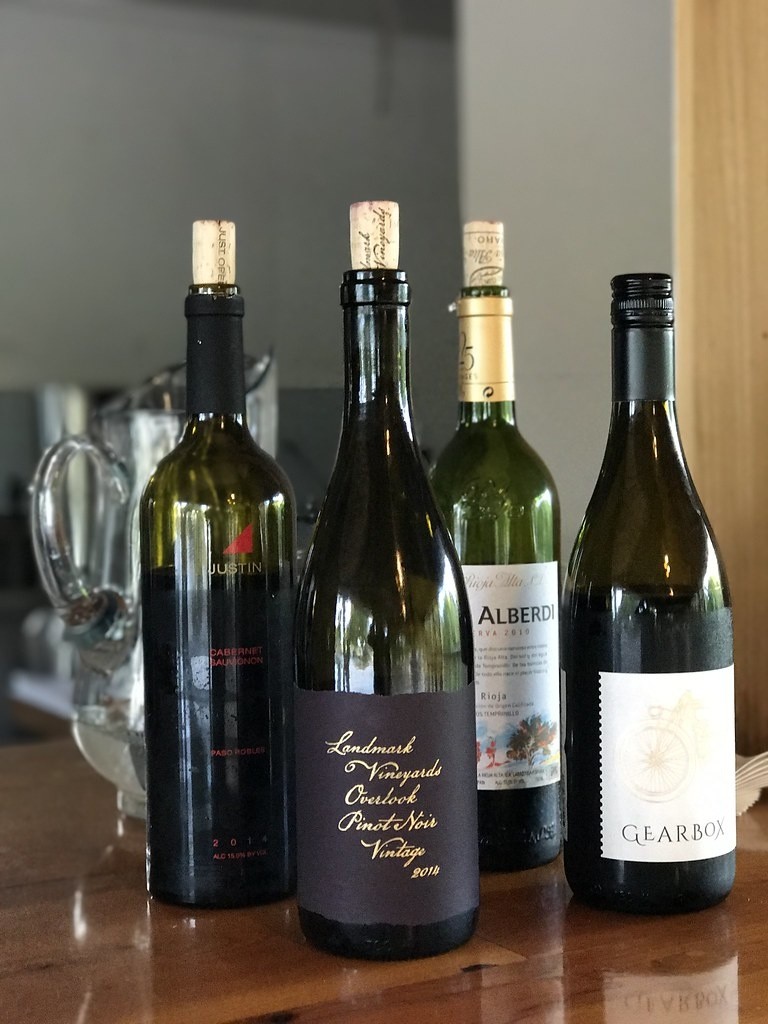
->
[735,751,768,817]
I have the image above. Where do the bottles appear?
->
[428,220,562,873]
[291,202,478,958]
[140,219,298,909]
[560,274,735,913]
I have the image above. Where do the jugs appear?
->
[30,349,276,821]
